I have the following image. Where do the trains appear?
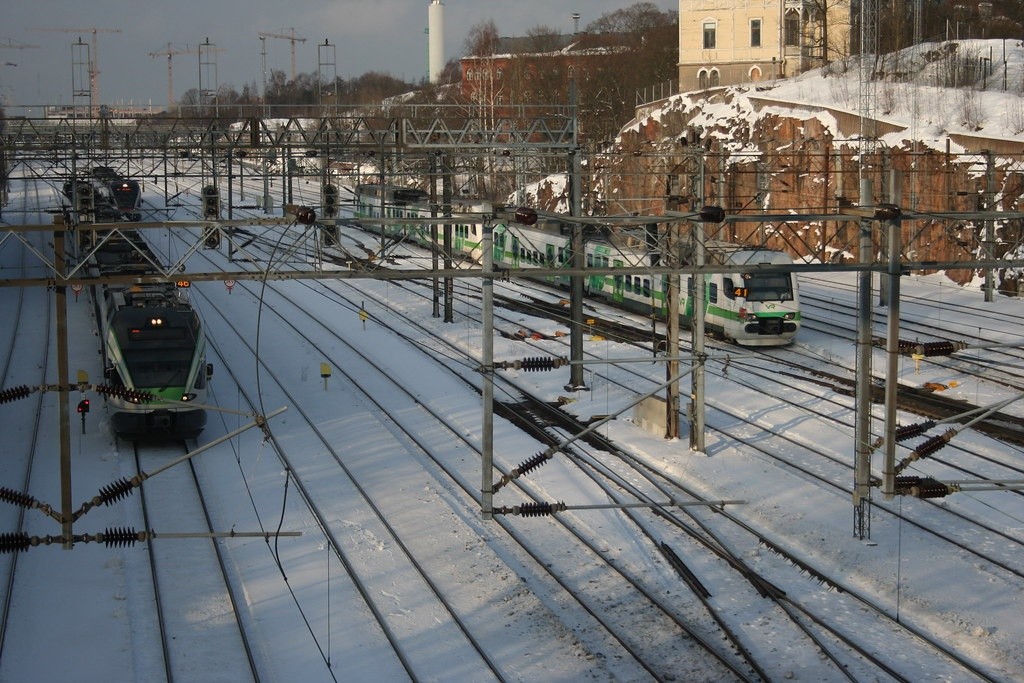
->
[62,180,214,443]
[90,165,143,221]
[350,183,803,349]
